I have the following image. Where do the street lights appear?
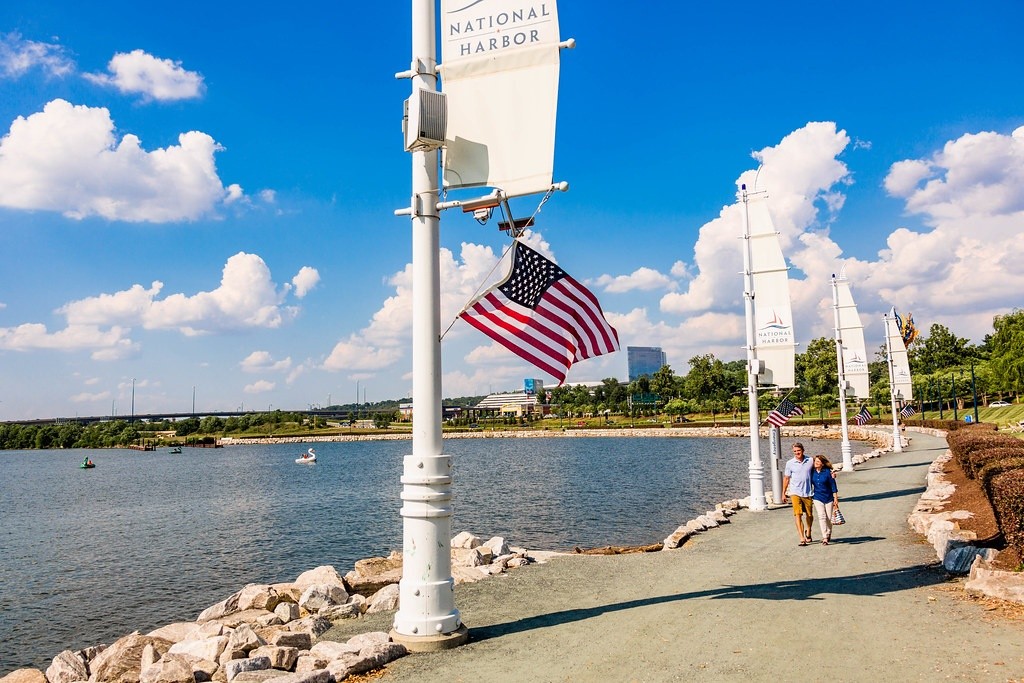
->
[237,406,241,418]
[242,402,244,411]
[328,393,331,406]
[192,385,195,413]
[269,403,272,415]
[131,378,137,429]
[308,403,315,409]
[356,379,359,403]
[363,383,366,403]
[317,403,320,409]
[112,399,115,416]
[115,407,117,415]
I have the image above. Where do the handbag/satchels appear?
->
[830,503,846,525]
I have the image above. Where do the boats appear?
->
[295,447,317,465]
[80,455,96,468]
[168,446,183,453]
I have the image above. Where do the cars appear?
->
[521,423,529,427]
[578,421,588,425]
[603,419,619,426]
[988,400,1013,408]
[470,423,480,428]
[675,417,696,422]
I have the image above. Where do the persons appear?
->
[782,443,836,546]
[901,423,906,435]
[822,424,828,429]
[89,460,92,464]
[300,454,308,459]
[812,454,838,544]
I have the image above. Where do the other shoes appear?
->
[821,534,831,545]
[798,539,805,546]
[805,530,812,543]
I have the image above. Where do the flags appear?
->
[461,240,620,387]
[901,405,916,417]
[766,398,806,428]
[854,405,872,426]
